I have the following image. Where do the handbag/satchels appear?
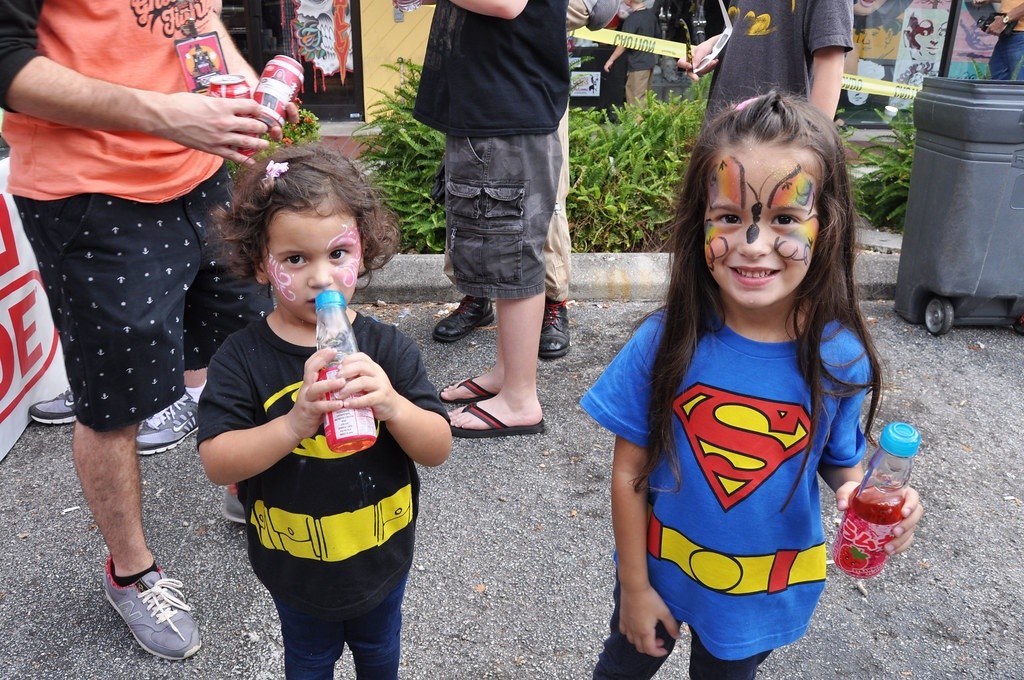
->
[982,12,1018,37]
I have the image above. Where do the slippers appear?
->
[438,376,497,403]
[449,403,542,438]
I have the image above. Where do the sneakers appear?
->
[29,388,78,424]
[221,484,246,524]
[104,556,202,660]
[135,391,198,455]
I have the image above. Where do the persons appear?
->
[973,0,1024,81]
[0,0,300,661]
[578,88,922,680]
[676,0,854,131]
[396,0,621,438]
[603,0,664,118]
[196,140,451,680]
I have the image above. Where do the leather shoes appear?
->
[433,295,493,341]
[538,297,570,357]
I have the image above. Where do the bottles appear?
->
[397,0,422,12]
[314,291,376,454]
[832,422,920,580]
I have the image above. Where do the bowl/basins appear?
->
[885,106,898,116]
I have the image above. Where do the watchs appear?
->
[1002,13,1013,25]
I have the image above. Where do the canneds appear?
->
[249,55,304,130]
[207,74,261,156]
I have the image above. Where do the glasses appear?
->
[679,0,733,73]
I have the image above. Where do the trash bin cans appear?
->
[893,78,1024,336]
